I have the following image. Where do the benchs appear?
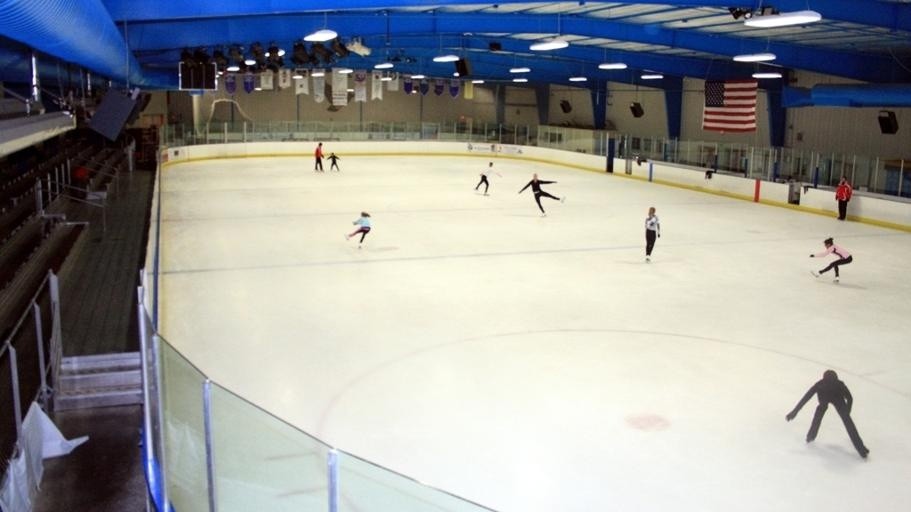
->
[0,138,129,403]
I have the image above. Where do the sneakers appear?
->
[811,270,839,282]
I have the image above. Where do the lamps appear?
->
[210,45,286,81]
[508,65,532,86]
[732,0,823,80]
[568,75,588,84]
[528,38,572,54]
[640,70,663,84]
[597,60,628,73]
[302,27,339,44]
[290,53,486,92]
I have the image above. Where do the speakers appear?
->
[454,58,472,79]
[559,101,571,113]
[630,102,644,118]
[88,87,141,142]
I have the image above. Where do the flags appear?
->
[702,79,758,135]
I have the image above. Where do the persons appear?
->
[328,152,342,172]
[786,370,870,457]
[457,116,470,141]
[315,142,324,171]
[706,149,715,168]
[519,174,564,216]
[76,98,93,127]
[346,212,372,248]
[809,236,854,282]
[645,207,662,261]
[476,161,502,196]
[834,176,854,220]
[63,96,76,115]
